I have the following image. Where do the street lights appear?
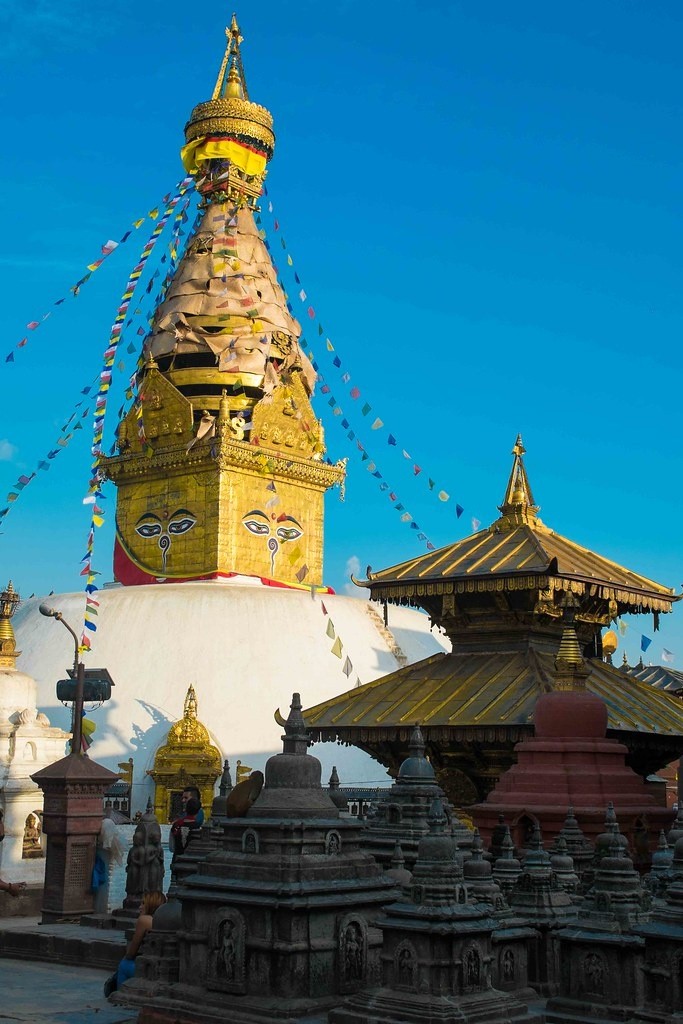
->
[40,603,79,744]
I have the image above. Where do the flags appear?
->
[2,163,673,756]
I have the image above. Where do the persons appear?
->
[633,811,651,855]
[178,786,204,829]
[505,954,514,977]
[347,931,361,980]
[219,928,235,982]
[125,831,144,902]
[586,960,602,993]
[115,890,166,991]
[468,955,478,985]
[146,832,163,893]
[23,818,41,850]
[0,879,27,897]
[402,953,412,985]
[171,798,201,865]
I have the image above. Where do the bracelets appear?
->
[6,882,11,892]
